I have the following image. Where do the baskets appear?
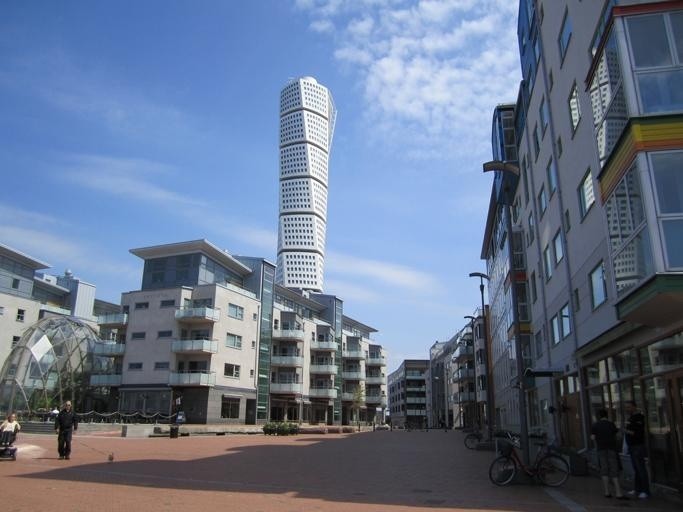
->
[499,439,515,457]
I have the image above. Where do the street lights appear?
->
[481,159,530,477]
[432,314,478,430]
[468,272,492,449]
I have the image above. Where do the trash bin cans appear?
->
[169,424,178,438]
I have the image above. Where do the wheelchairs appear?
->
[0,427,17,460]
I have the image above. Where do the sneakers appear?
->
[605,489,649,499]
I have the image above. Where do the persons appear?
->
[588,409,625,499]
[0,412,19,445]
[55,400,79,459]
[617,399,649,499]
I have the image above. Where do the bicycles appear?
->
[462,419,502,450]
[488,430,569,487]
